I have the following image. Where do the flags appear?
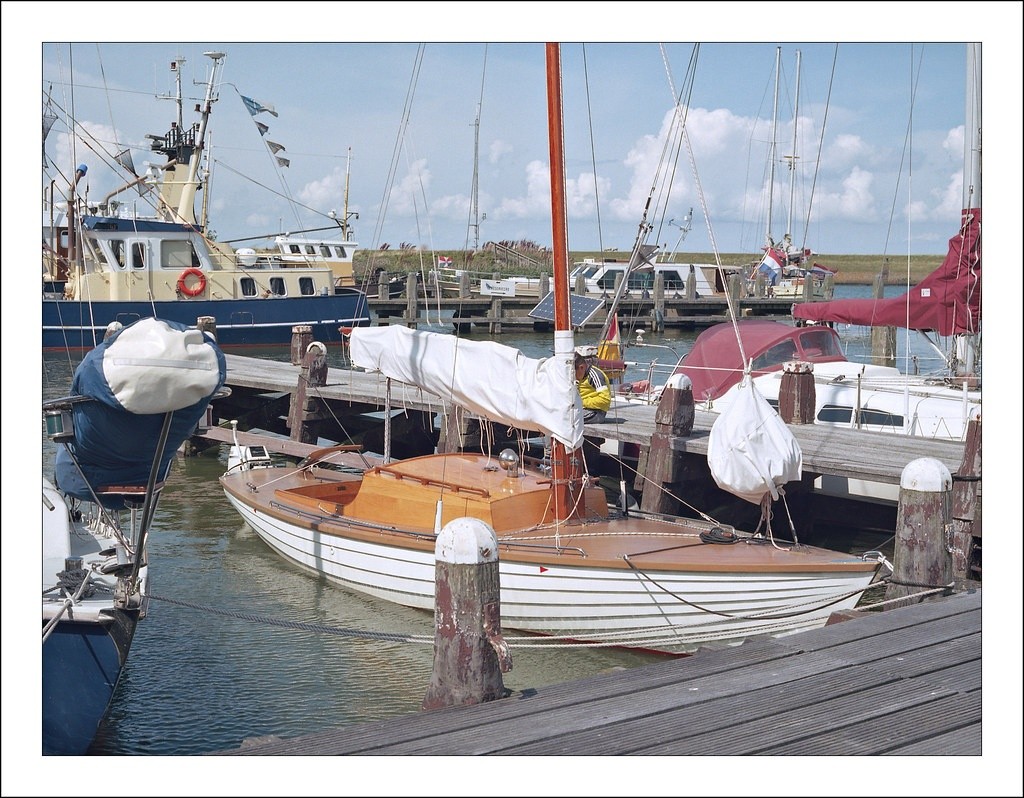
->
[254,121,270,136]
[266,141,285,154]
[438,257,454,269]
[275,156,290,168]
[240,95,278,117]
[757,247,783,286]
[810,263,838,280]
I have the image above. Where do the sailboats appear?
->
[45,40,982,659]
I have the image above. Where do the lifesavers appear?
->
[178,268,206,297]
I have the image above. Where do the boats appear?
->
[43,316,226,756]
